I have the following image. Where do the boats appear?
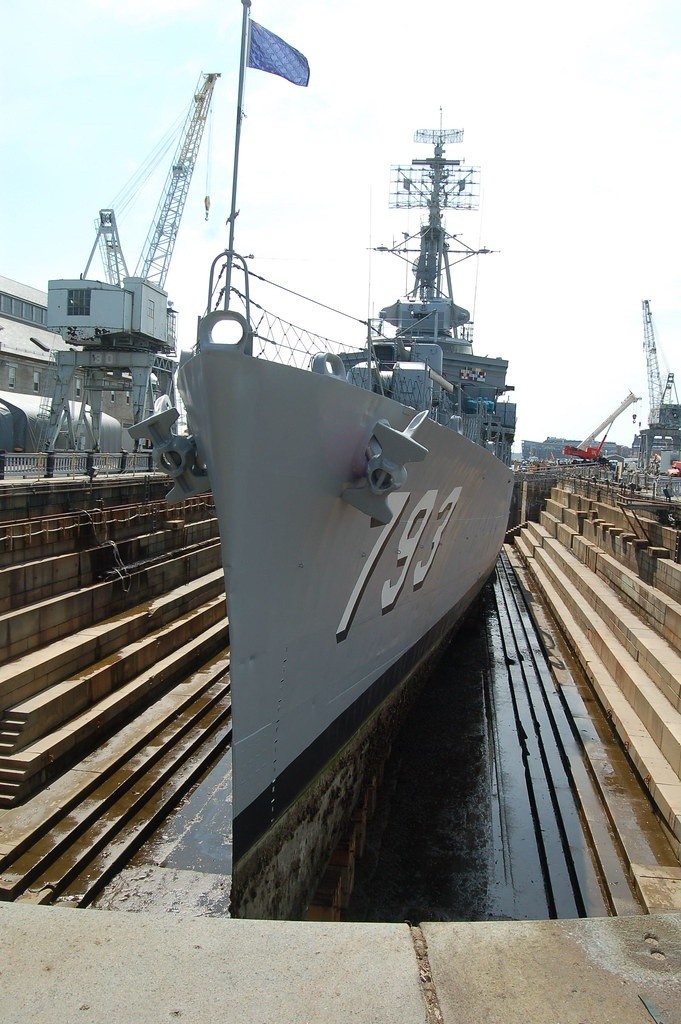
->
[180,117,521,922]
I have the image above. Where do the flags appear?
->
[245,18,310,88]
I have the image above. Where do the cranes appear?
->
[42,71,224,353]
[640,299,681,429]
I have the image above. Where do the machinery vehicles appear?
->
[563,394,643,464]
